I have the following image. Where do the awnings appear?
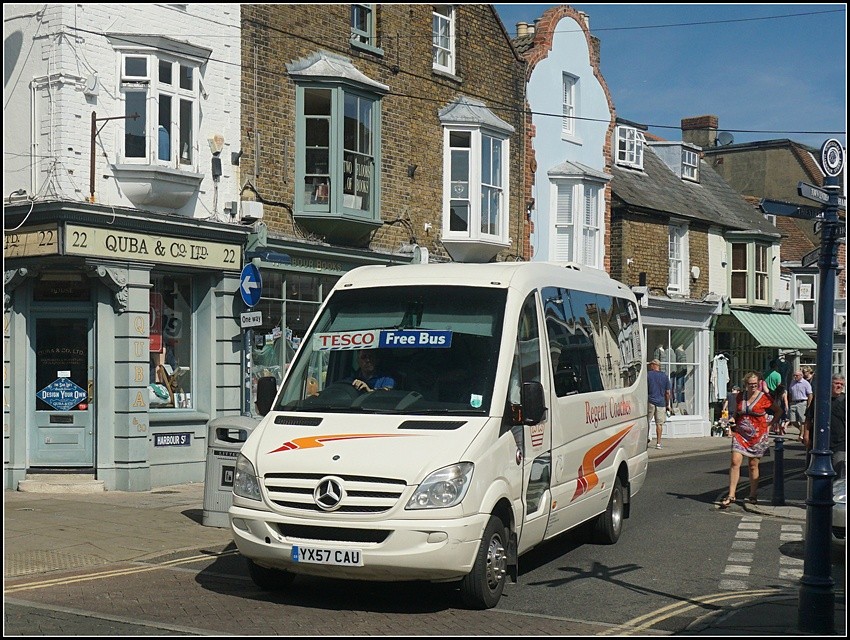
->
[731,310,817,349]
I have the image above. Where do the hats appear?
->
[649,358,661,366]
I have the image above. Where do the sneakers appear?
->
[657,442,663,449]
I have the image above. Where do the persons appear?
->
[647,359,672,449]
[351,350,394,393]
[721,372,783,507]
[722,360,814,440]
[804,374,845,482]
[654,343,687,403]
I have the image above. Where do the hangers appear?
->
[711,353,729,362]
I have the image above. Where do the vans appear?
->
[227,256,652,609]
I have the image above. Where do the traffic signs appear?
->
[835,225,847,238]
[838,195,847,210]
[757,198,826,222]
[798,183,832,205]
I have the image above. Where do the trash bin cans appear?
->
[201,416,260,528]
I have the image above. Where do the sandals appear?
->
[720,495,736,505]
[749,496,757,504]
